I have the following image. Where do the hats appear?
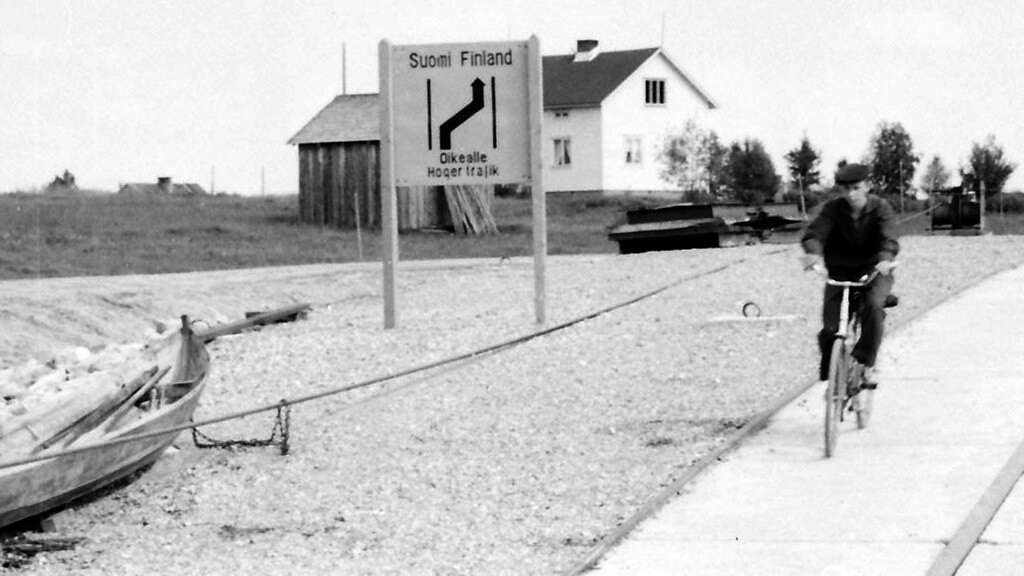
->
[835,164,870,184]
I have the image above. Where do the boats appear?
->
[0,310,213,533]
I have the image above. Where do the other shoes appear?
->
[861,366,877,386]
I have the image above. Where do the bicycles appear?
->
[809,262,898,462]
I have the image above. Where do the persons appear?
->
[798,163,901,404]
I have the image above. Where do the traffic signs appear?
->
[395,40,529,188]
